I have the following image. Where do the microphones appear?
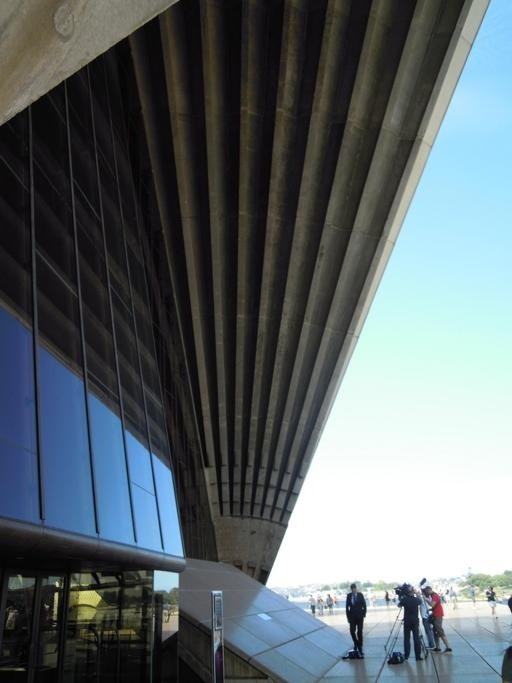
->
[419,578,426,587]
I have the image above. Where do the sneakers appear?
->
[433,648,441,651]
[444,648,452,651]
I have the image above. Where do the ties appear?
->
[353,595,355,602]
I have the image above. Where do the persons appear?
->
[422,585,452,653]
[396,587,424,660]
[345,584,367,656]
[501,596,512,683]
[308,594,335,617]
[383,584,509,615]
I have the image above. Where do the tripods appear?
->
[384,608,429,658]
